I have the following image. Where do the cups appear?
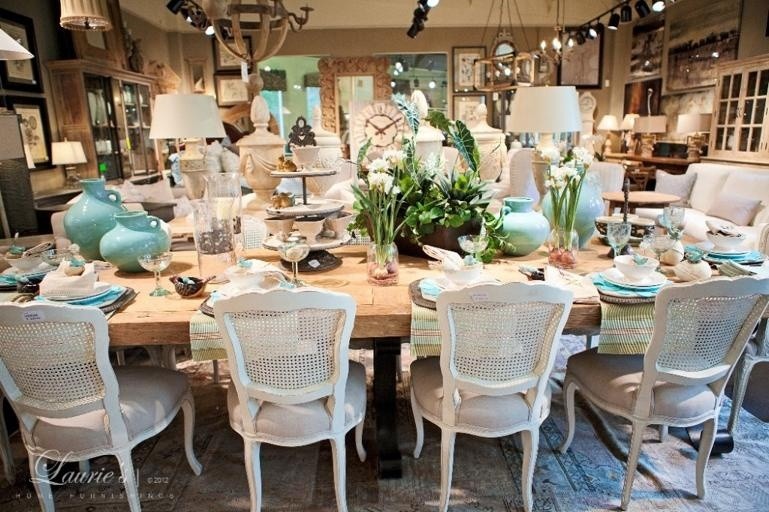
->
[663,206,685,239]
[137,254,174,297]
[548,229,579,271]
[277,243,311,291]
[200,172,244,254]
[457,234,490,269]
[643,235,677,275]
[190,197,239,284]
[607,221,631,271]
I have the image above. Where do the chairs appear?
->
[562,276,765,511]
[410,281,573,511]
[0,300,203,512]
[215,288,368,512]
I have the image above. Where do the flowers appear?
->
[341,146,443,267]
[544,157,590,268]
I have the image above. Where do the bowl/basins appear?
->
[264,217,295,243]
[324,211,353,239]
[705,231,747,251]
[290,146,321,171]
[167,275,216,298]
[614,255,660,282]
[293,217,326,245]
[223,270,265,290]
[441,264,484,285]
[4,256,46,270]
[595,216,656,239]
[40,249,64,266]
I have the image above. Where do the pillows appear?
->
[705,194,762,227]
[655,169,697,208]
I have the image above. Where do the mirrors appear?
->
[317,54,390,135]
[489,30,520,57]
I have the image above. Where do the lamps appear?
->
[621,113,639,154]
[634,0,651,17]
[51,142,86,190]
[677,113,712,162]
[607,13,621,30]
[509,84,582,213]
[651,0,665,14]
[149,91,226,202]
[597,115,620,155]
[588,23,603,38]
[575,30,586,45]
[620,5,632,22]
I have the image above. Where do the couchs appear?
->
[613,162,769,256]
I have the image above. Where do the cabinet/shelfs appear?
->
[46,61,168,189]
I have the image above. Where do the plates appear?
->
[710,250,751,255]
[701,254,765,265]
[45,281,110,301]
[600,269,668,290]
[707,248,751,258]
[0,287,16,291]
[419,279,502,303]
[33,284,128,308]
[603,271,666,287]
[33,286,120,304]
[592,272,665,292]
[0,275,17,286]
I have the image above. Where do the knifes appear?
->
[518,268,545,280]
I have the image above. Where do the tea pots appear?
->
[491,197,551,256]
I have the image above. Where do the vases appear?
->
[545,226,579,270]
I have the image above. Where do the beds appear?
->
[0,249,769,478]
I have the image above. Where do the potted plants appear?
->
[396,160,507,260]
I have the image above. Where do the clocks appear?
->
[578,90,597,120]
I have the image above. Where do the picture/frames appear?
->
[451,93,486,124]
[560,29,603,89]
[453,44,485,93]
[4,94,53,170]
[213,35,254,73]
[0,9,43,92]
[623,78,665,132]
[72,0,122,66]
[214,73,252,107]
[629,15,664,74]
[667,0,744,89]
